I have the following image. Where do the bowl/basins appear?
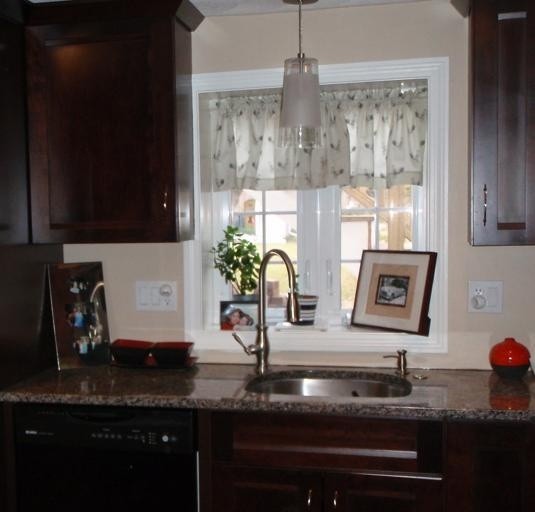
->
[109,333,191,365]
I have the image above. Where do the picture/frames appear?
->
[48,261,111,373]
[348,248,441,336]
[219,300,261,333]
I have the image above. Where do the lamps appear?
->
[275,0,328,154]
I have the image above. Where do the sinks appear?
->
[244,363,413,398]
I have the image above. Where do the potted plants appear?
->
[207,223,262,300]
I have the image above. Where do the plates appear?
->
[107,355,199,374]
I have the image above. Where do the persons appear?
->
[64,300,103,363]
[221,308,244,331]
[232,314,255,332]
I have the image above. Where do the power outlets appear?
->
[134,280,179,314]
[467,281,503,313]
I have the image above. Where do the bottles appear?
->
[489,335,532,380]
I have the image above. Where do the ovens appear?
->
[0,403,202,511]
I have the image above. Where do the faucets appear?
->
[232,249,299,372]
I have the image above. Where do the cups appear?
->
[296,294,319,325]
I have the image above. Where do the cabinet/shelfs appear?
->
[467,0,535,248]
[0,0,206,247]
[0,409,535,512]
